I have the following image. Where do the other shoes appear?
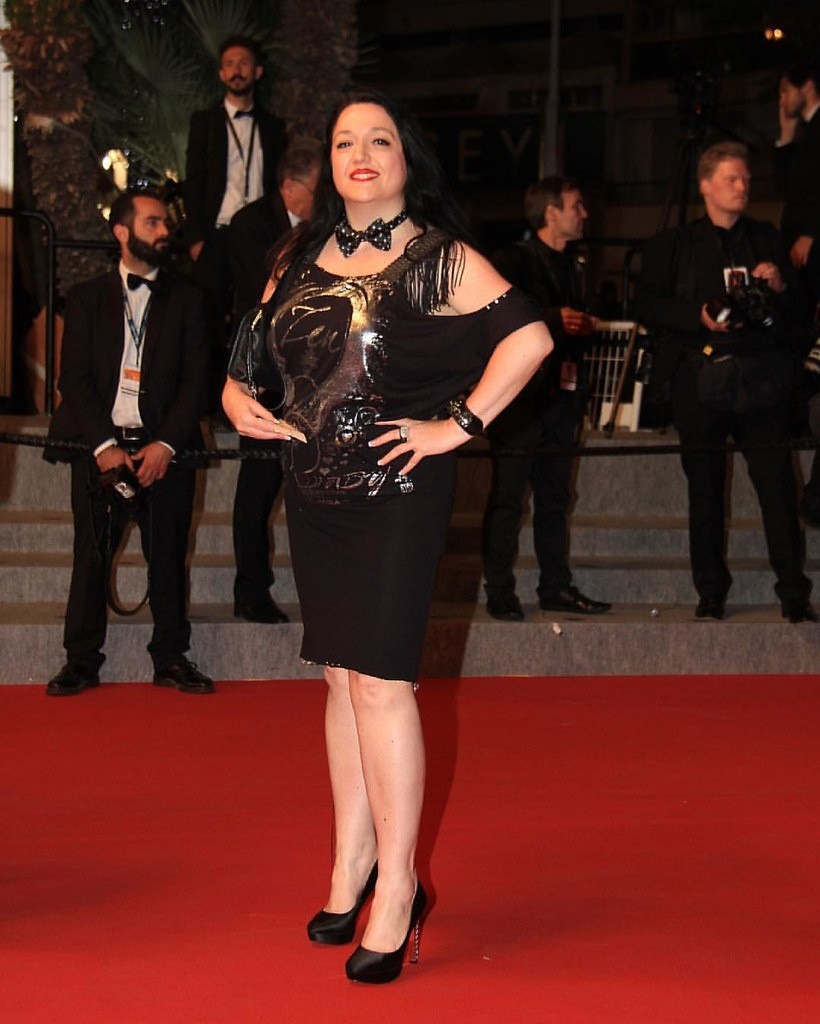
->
[695,596,727,621]
[782,602,820,623]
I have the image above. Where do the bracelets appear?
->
[447,395,484,436]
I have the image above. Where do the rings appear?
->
[399,426,408,442]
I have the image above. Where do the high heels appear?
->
[346,879,429,983]
[307,859,379,945]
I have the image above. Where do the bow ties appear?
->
[234,109,257,118]
[127,273,169,298]
[333,208,408,258]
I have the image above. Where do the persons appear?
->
[480,177,613,621]
[185,35,289,261]
[215,136,321,623]
[771,61,820,531]
[218,89,554,984]
[42,187,216,695]
[626,142,820,624]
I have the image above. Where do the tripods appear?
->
[655,113,758,237]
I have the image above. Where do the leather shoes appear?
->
[539,592,612,614]
[233,599,291,624]
[46,665,100,695]
[486,600,524,620]
[152,655,217,694]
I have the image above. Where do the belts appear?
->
[112,425,146,441]
